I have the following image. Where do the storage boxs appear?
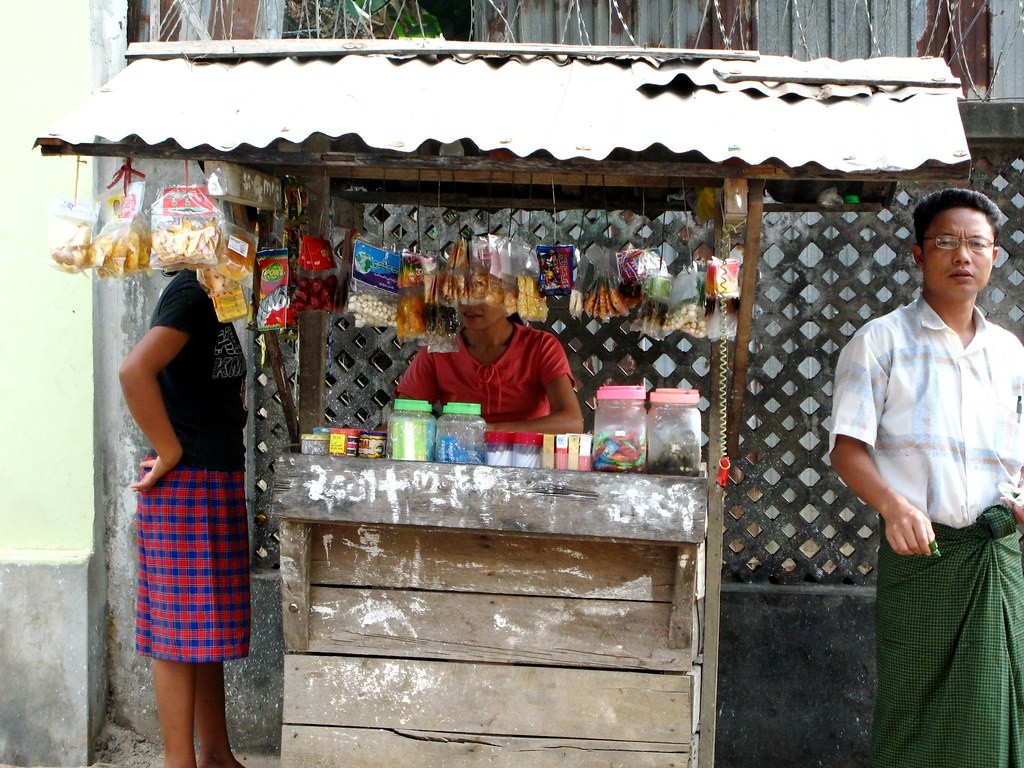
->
[555,434,569,470]
[568,435,580,470]
[578,434,592,471]
[543,434,556,469]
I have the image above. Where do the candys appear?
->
[593,430,647,473]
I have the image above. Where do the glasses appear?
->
[922,235,994,250]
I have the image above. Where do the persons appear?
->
[393,261,584,453]
[118,269,252,768]
[825,186,1023,768]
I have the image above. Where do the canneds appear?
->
[330,428,387,459]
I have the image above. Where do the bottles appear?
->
[486,431,512,467]
[386,399,437,462]
[437,402,485,467]
[593,385,648,474]
[513,433,544,470]
[329,428,360,457]
[647,387,701,476]
[357,431,387,460]
[301,433,329,456]
